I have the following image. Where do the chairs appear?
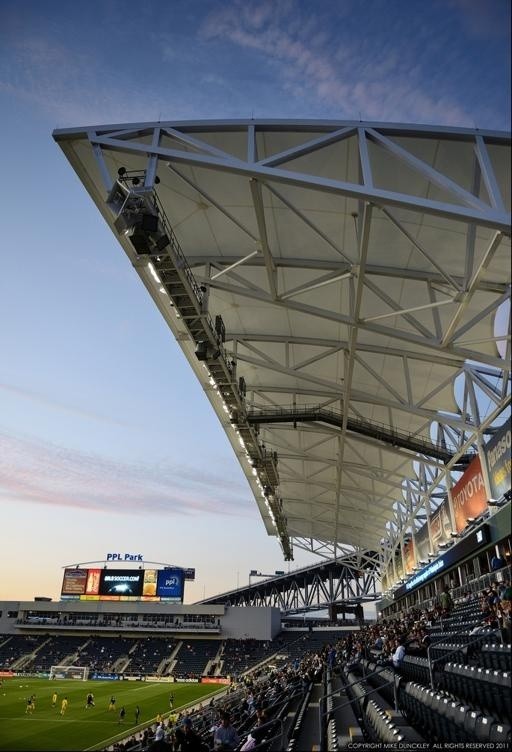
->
[235,598,510,752]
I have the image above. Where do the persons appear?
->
[0,578,512,752]
